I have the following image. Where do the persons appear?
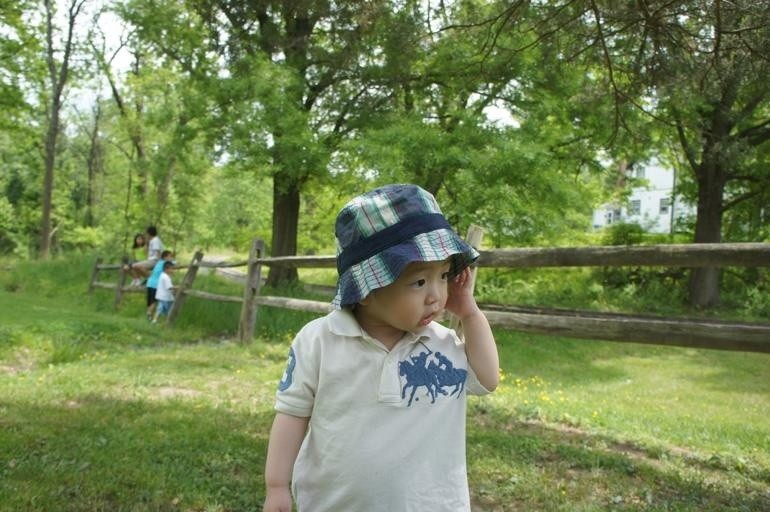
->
[151,261,179,323]
[132,226,165,283]
[263,183,499,512]
[123,234,148,286]
[145,250,173,322]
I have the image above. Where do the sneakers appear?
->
[131,277,145,286]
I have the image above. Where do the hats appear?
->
[330,183,480,311]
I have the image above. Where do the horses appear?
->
[398,360,448,407]
[426,360,467,398]
